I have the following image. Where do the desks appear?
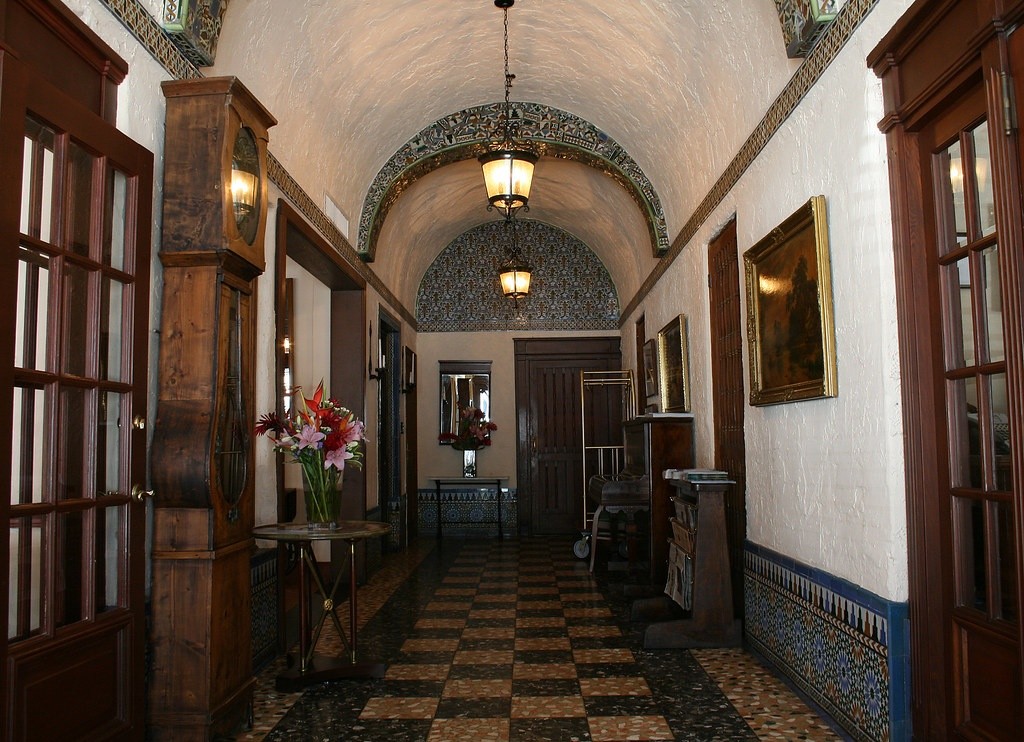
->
[426,477,509,544]
[246,521,394,673]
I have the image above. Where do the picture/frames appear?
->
[641,338,658,400]
[656,313,692,414]
[742,194,839,406]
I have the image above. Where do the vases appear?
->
[301,464,339,532]
[463,450,477,478]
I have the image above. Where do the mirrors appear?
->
[437,360,493,446]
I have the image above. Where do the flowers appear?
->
[437,400,498,451]
[252,376,370,522]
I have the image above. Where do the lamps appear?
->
[497,209,534,310]
[477,0,540,236]
[231,143,260,230]
[284,337,289,354]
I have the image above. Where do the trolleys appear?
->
[573,368,636,558]
